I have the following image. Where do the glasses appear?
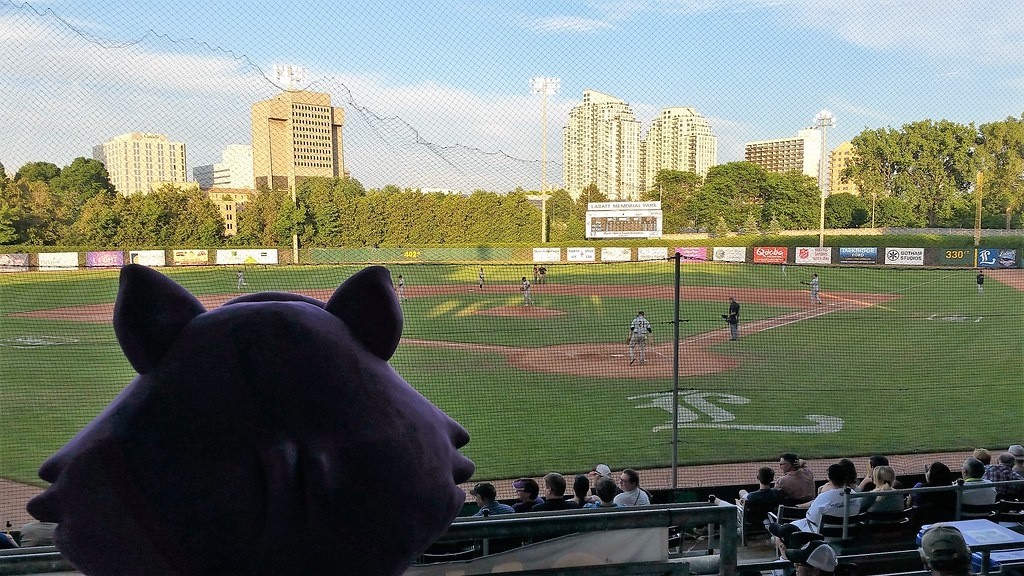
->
[619,477,636,483]
[779,463,787,466]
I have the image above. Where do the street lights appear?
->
[529,76,561,242]
[274,64,306,261]
[870,192,877,228]
[812,108,837,247]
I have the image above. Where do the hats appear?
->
[593,463,611,478]
[768,522,802,545]
[785,540,838,573]
[470,482,496,497]
[921,525,972,561]
[512,478,525,491]
[1008,445,1024,460]
[397,275,402,278]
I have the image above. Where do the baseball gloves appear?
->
[626,337,632,345]
[520,287,524,291]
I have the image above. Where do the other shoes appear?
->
[692,527,708,536]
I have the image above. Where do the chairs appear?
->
[733,498,1024,559]
[669,523,686,558]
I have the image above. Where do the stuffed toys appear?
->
[25,264,476,576]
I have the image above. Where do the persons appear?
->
[521,276,535,306]
[237,270,249,289]
[0,532,19,549]
[396,274,408,304]
[808,274,821,305]
[977,270,984,293]
[511,463,652,514]
[21,521,59,547]
[470,482,515,516]
[727,295,740,341]
[479,268,485,291]
[629,311,652,365]
[736,445,1024,576]
[781,258,787,275]
[534,265,547,284]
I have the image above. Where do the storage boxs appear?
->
[915,518,1024,573]
